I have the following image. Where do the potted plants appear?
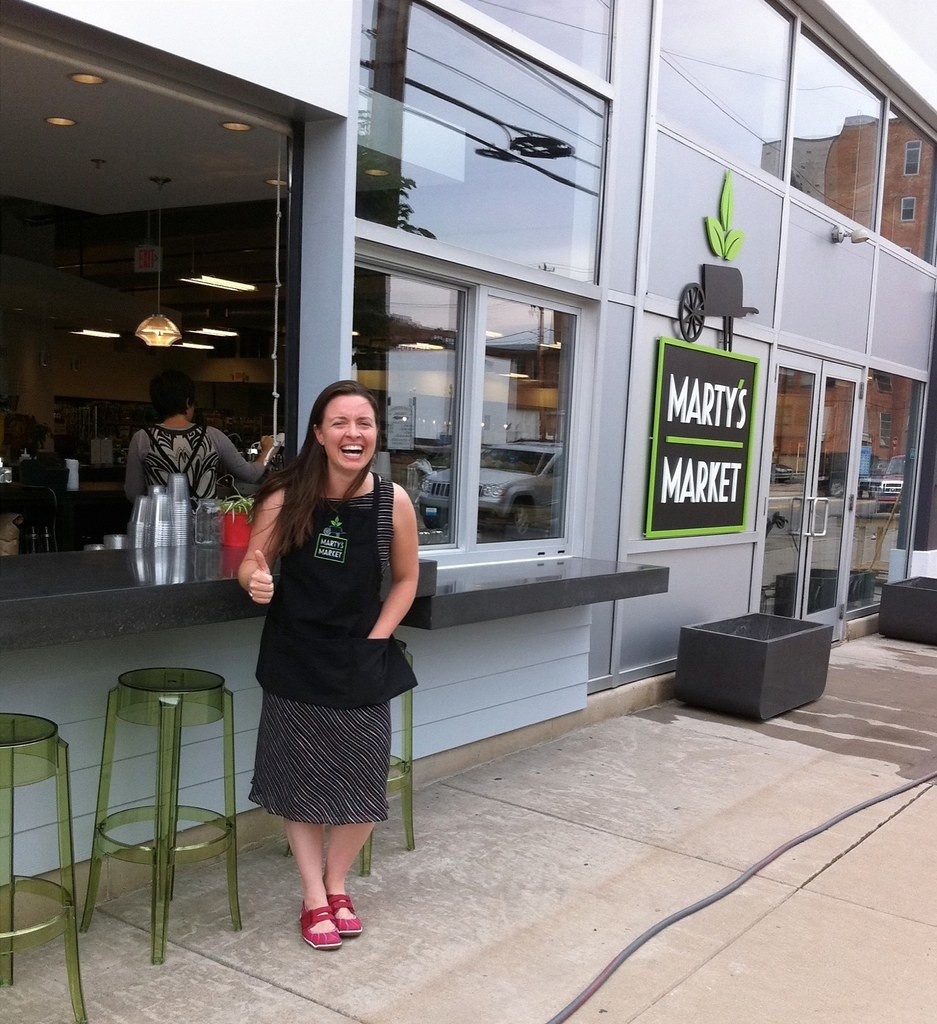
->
[218,494,254,546]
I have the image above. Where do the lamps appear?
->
[63,175,259,351]
[830,224,869,244]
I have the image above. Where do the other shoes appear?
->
[327,894,362,936]
[300,899,342,950]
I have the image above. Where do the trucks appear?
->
[818,446,879,497]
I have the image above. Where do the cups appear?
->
[84,473,192,550]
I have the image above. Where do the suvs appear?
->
[418,439,563,536]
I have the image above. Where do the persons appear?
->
[125,369,282,503]
[237,380,420,951]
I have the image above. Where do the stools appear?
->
[0,712,87,1024]
[79,667,242,964]
[284,639,414,877]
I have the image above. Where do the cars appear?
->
[771,462,793,482]
[877,455,906,511]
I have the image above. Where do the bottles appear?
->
[19,448,31,462]
[195,499,215,544]
[41,525,51,552]
[28,526,40,554]
